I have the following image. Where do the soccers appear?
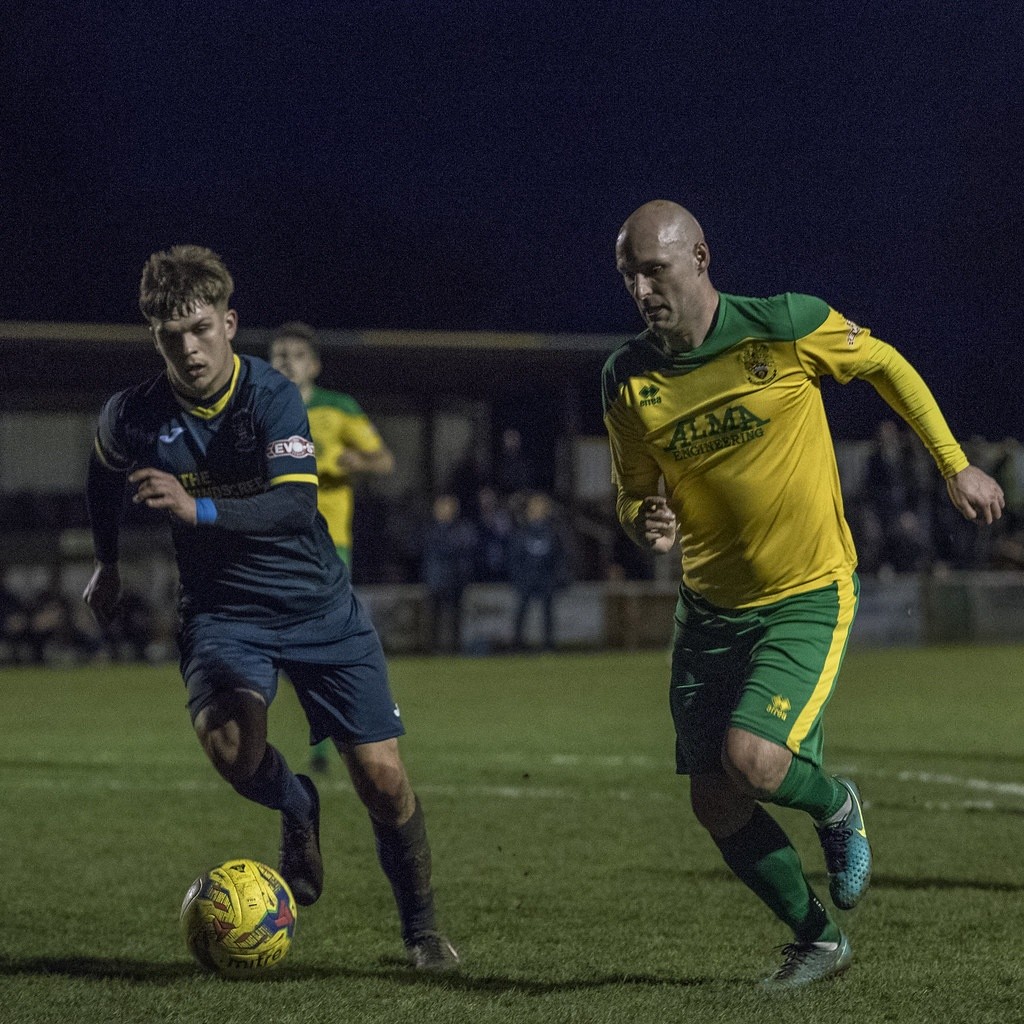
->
[179,858,297,975]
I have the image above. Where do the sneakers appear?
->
[764,927,852,989]
[278,774,324,906]
[403,932,459,972]
[813,775,873,911]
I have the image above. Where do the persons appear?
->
[270,322,398,777]
[351,427,654,582]
[505,494,570,648]
[81,243,463,980]
[421,491,472,652]
[3,530,178,668]
[601,199,1006,992]
[832,415,1024,584]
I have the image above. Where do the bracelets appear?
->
[193,496,219,525]
[94,543,121,564]
